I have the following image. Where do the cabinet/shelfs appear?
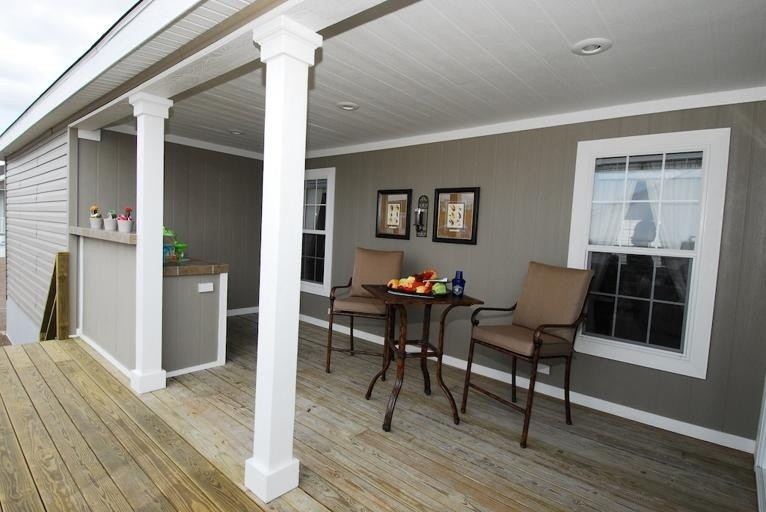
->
[163,253,230,380]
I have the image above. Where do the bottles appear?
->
[451,271,466,296]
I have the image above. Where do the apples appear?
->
[388,271,446,294]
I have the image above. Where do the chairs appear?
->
[461,262,594,446]
[328,246,404,380]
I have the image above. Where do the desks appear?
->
[362,284,484,432]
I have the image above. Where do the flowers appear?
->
[88,205,132,219]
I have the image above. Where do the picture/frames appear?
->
[375,187,480,245]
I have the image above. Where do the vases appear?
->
[89,217,134,233]
